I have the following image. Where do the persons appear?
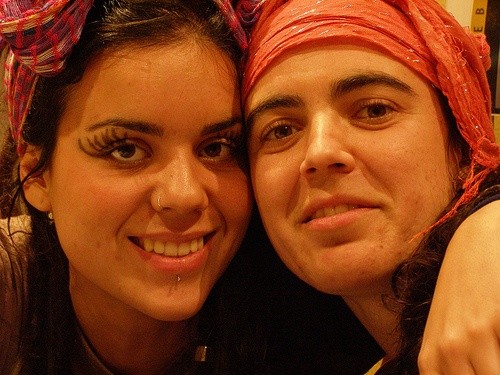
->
[241,0,500,375]
[0,1,500,375]
